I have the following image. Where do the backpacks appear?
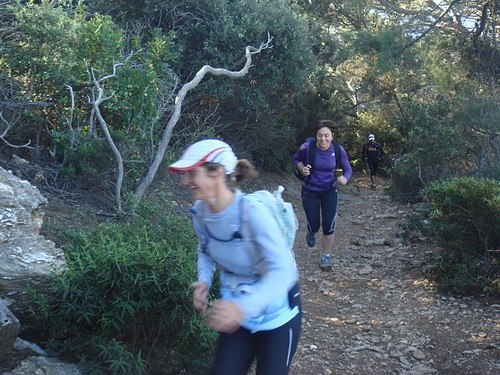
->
[240,185,299,277]
[293,137,342,181]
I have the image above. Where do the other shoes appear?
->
[372,183,376,189]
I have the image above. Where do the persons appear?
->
[169,139,302,375]
[363,134,384,187]
[293,120,352,271]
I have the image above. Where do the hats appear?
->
[368,134,375,141]
[166,139,239,175]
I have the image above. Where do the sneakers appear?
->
[306,231,315,247]
[319,251,333,271]
